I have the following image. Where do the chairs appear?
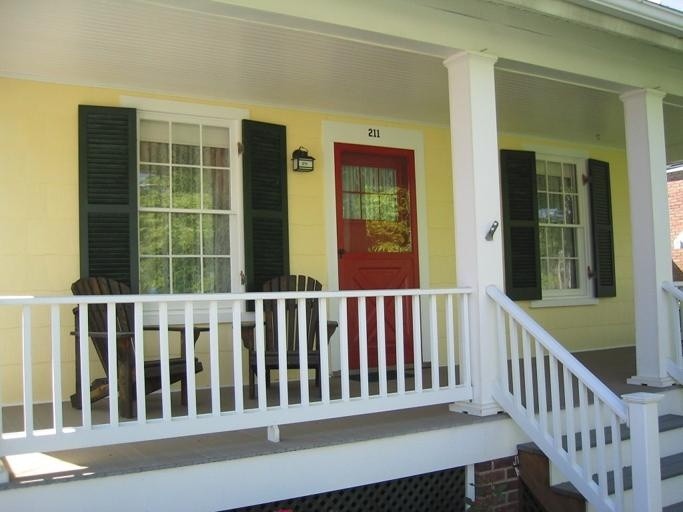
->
[68,277,205,419]
[233,275,338,399]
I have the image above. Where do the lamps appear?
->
[291,146,316,173]
